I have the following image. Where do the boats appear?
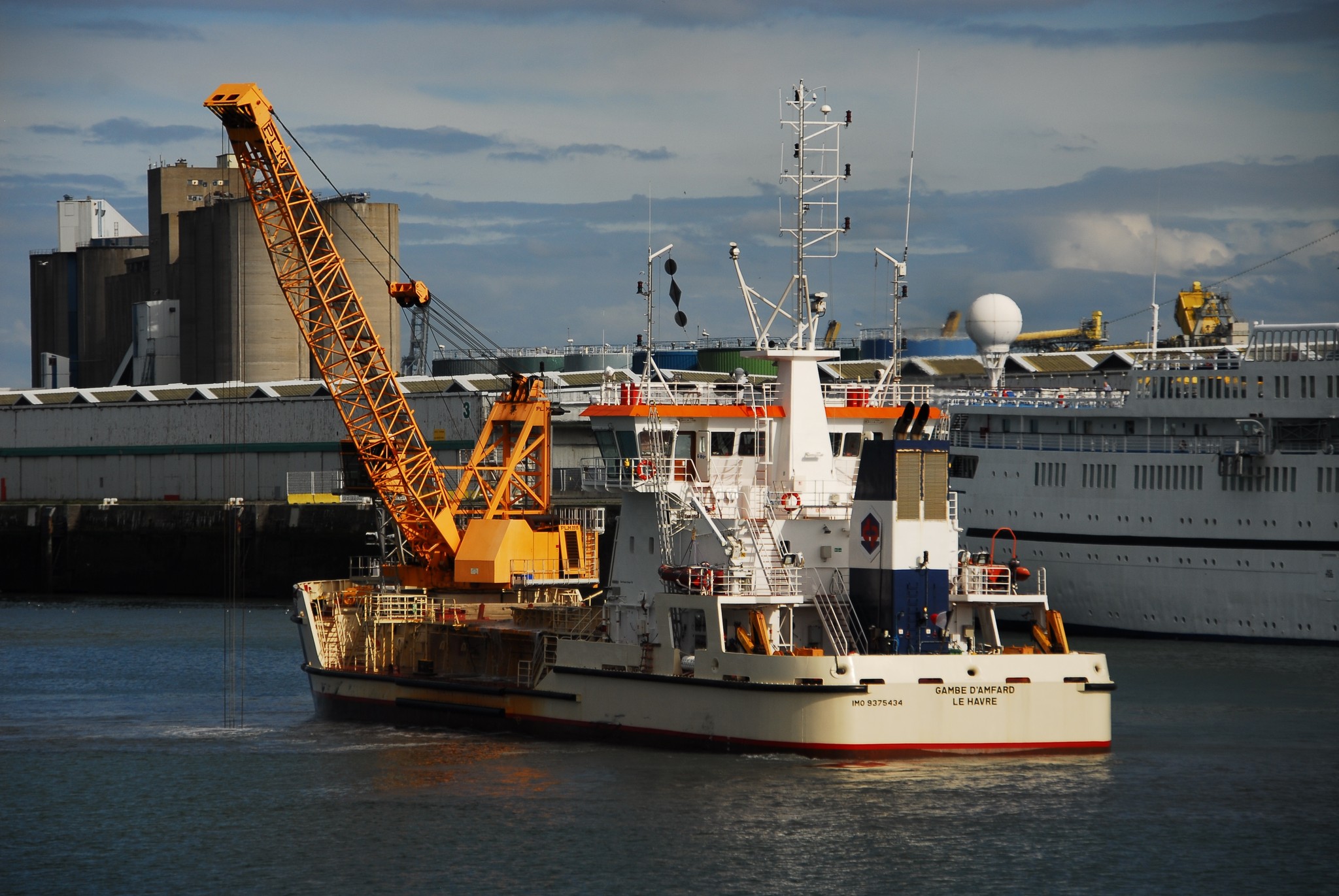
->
[200,74,1123,757]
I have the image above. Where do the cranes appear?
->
[208,82,605,596]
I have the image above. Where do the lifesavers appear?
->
[781,492,801,512]
[344,593,356,606]
[702,570,713,591]
[637,460,656,479]
